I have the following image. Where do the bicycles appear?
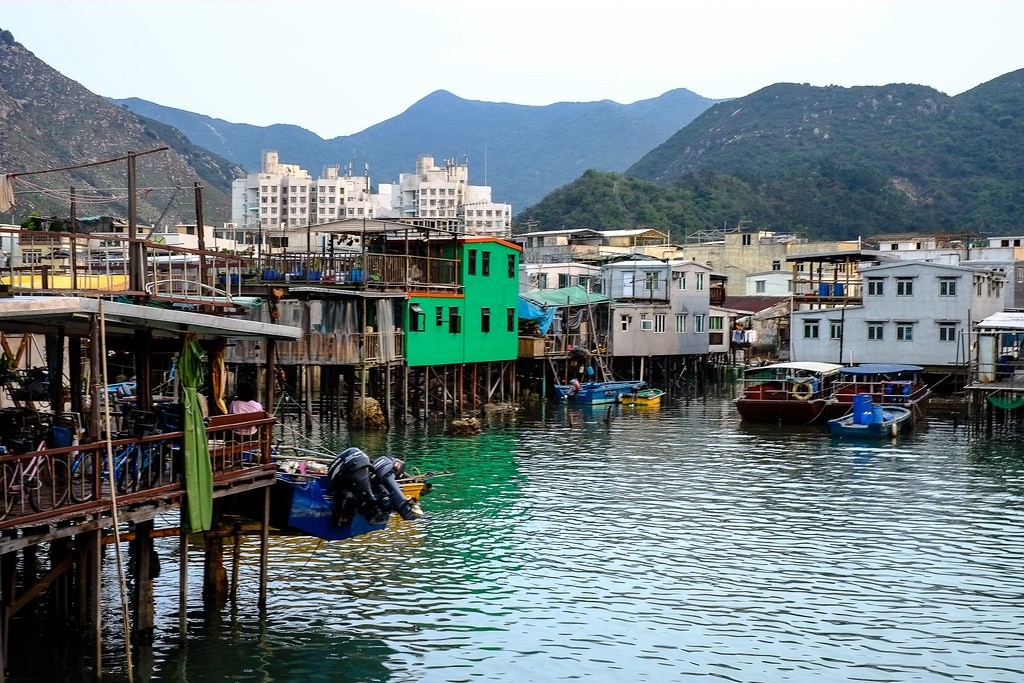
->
[0,408,68,522]
[69,407,158,505]
[131,410,210,494]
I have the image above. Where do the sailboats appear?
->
[167,368,426,542]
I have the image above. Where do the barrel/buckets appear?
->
[998,355,1015,378]
[853,393,883,425]
[579,363,594,375]
[887,384,911,403]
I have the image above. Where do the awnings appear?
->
[518,285,610,336]
[291,218,475,239]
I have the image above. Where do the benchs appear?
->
[170,438,262,476]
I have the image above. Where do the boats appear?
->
[621,389,663,406]
[825,363,933,428]
[730,361,847,424]
[828,406,912,440]
[554,381,646,405]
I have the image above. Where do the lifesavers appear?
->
[792,381,814,401]
[370,241,382,253]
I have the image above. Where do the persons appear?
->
[229,383,265,446]
[732,323,745,367]
